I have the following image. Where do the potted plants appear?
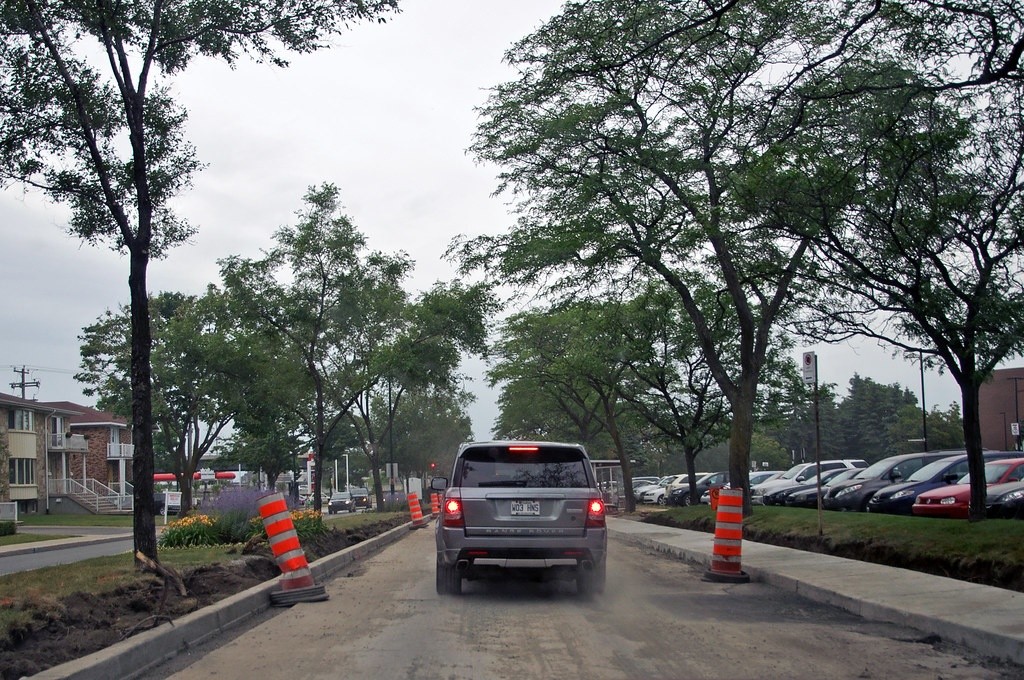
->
[65,432,73,439]
[84,434,91,439]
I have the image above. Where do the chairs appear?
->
[466,456,495,480]
[535,457,576,488]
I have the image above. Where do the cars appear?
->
[821,450,968,513]
[910,457,1024,519]
[632,450,969,513]
[967,478,1024,519]
[350,488,375,509]
[431,440,608,598]
[307,492,331,505]
[866,451,1024,515]
[328,492,357,514]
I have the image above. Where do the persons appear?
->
[303,494,313,510]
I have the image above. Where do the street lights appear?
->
[905,347,927,452]
[342,454,349,493]
[1007,377,1024,450]
